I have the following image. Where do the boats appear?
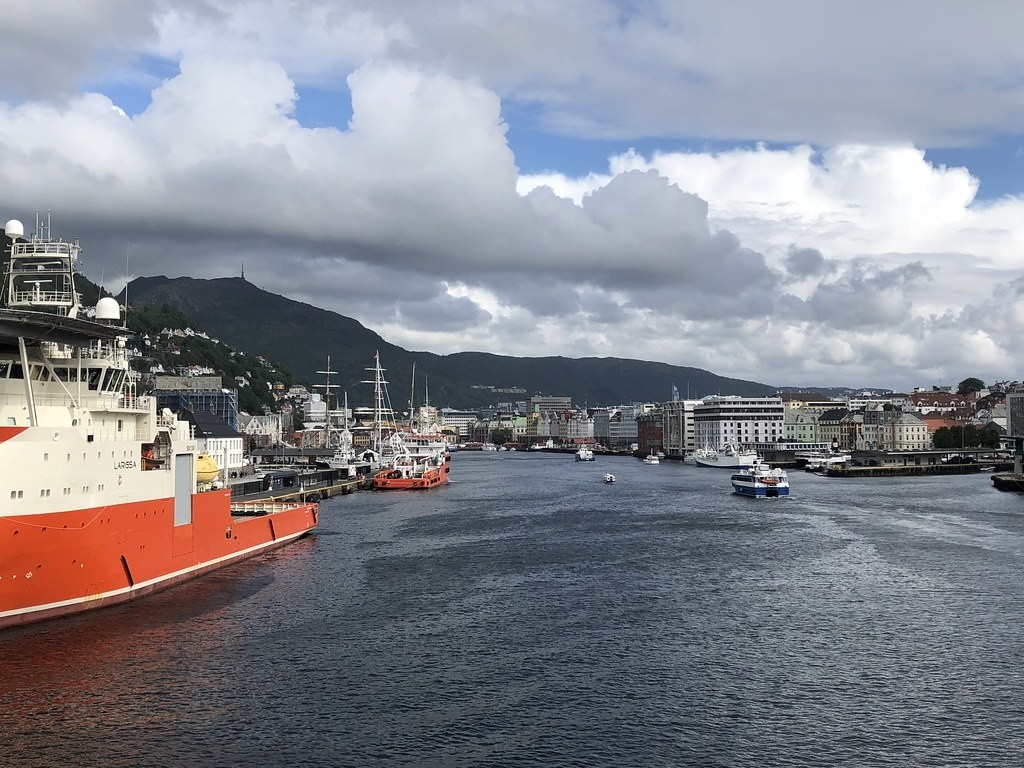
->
[693,435,759,469]
[730,461,789,498]
[803,464,825,473]
[574,442,596,462]
[371,360,453,490]
[643,453,660,465]
[480,442,498,451]
[603,472,616,484]
[0,207,320,630]
[655,451,666,459]
[193,450,219,483]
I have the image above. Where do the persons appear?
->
[146,449,155,469]
